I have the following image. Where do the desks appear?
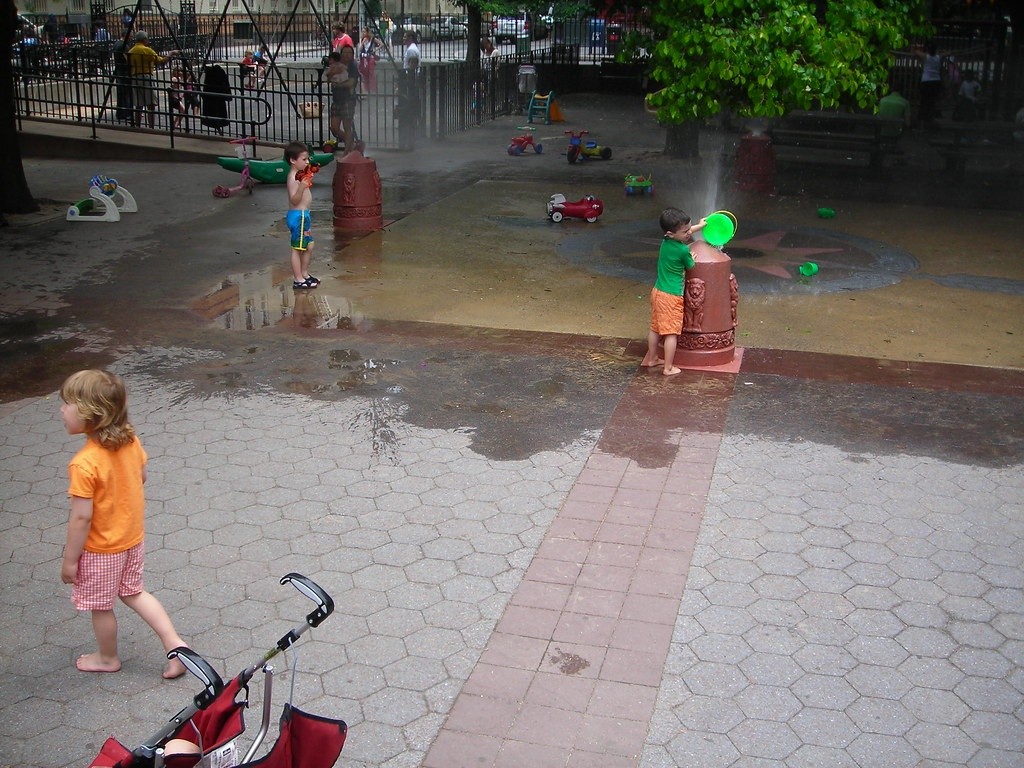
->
[767,109,904,179]
[934,119,1024,171]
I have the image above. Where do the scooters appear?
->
[210,137,259,198]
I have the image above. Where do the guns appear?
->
[295,162,322,189]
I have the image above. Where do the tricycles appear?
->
[564,130,612,163]
[506,126,543,156]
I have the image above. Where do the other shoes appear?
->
[350,94,356,98]
[258,79,261,83]
[331,104,335,110]
[261,78,264,81]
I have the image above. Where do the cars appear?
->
[351,9,531,44]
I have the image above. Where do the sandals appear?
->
[293,280,316,288]
[305,274,320,283]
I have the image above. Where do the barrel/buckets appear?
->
[692,210,737,246]
[802,262,818,276]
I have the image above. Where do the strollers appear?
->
[514,62,544,115]
[83,570,349,768]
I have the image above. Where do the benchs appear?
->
[762,127,896,154]
[928,139,1024,150]
[937,149,1024,161]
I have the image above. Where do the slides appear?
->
[535,94,563,120]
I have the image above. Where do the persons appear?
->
[647,206,708,376]
[19,20,508,160]
[58,369,188,678]
[284,140,321,289]
